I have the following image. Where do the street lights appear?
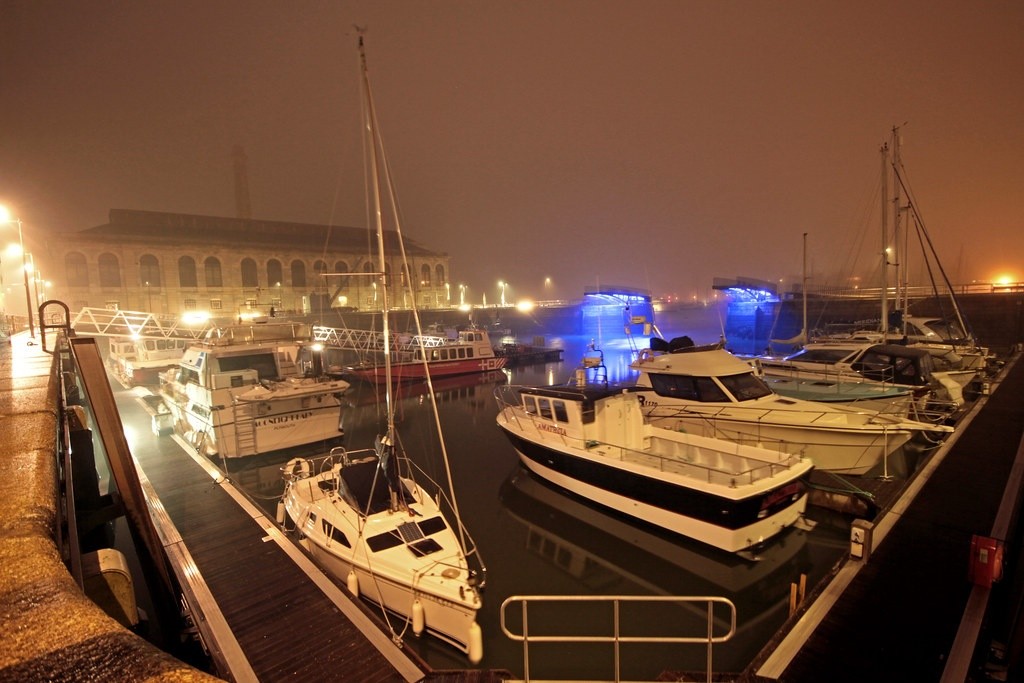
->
[498,280,505,306]
[0,213,34,337]
[6,243,43,311]
[459,284,464,307]
[544,277,551,288]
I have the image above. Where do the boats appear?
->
[494,338,815,556]
[499,470,821,635]
[624,316,957,476]
[108,310,510,463]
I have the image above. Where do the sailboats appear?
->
[273,22,488,667]
[734,120,1005,428]
